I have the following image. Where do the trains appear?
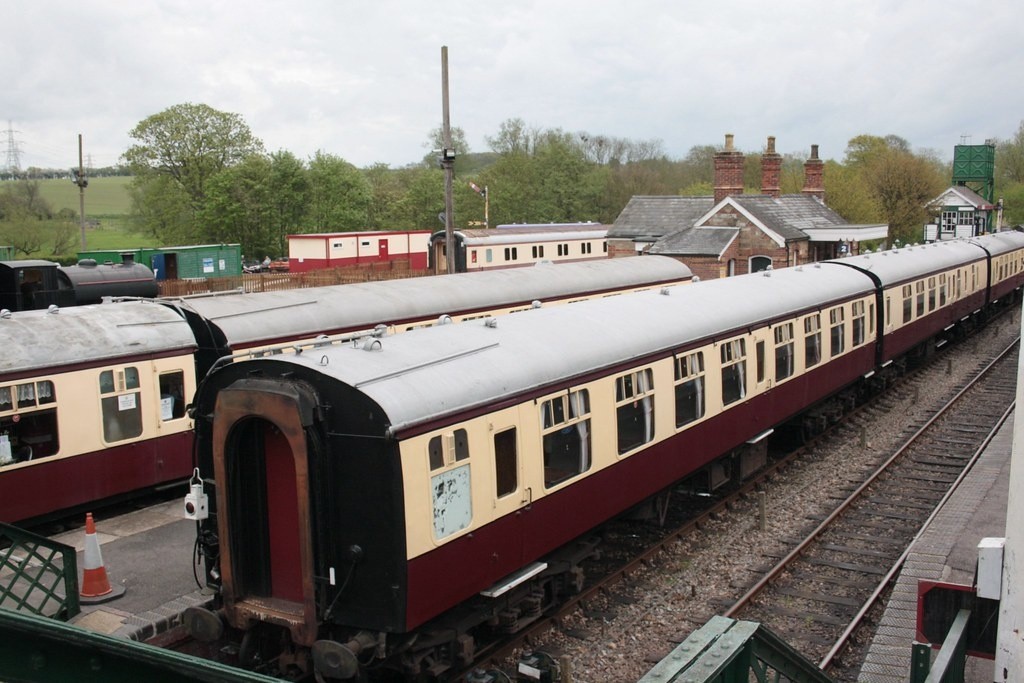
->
[0,251,161,317]
[0,256,694,525]
[184,224,1024,682]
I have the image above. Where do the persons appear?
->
[241,255,246,270]
[264,256,271,265]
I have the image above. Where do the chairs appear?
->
[16,445,32,463]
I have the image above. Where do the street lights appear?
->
[437,148,456,274]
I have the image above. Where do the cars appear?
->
[242,259,262,273]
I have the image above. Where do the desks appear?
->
[776,373,787,382]
[618,439,642,453]
[724,395,739,406]
[675,415,694,427]
[545,467,577,487]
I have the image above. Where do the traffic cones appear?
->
[78,512,126,606]
